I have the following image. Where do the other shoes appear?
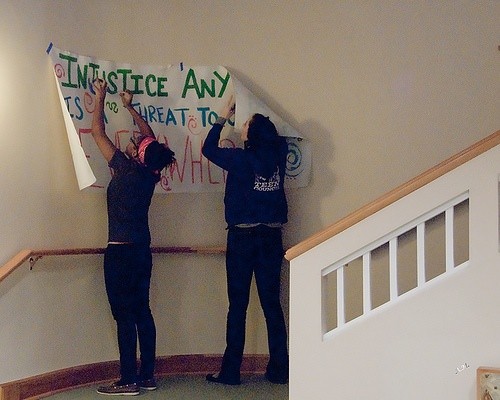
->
[206,373,222,383]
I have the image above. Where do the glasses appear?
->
[130,136,138,151]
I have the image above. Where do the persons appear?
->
[200,95,289,386]
[90,78,175,395]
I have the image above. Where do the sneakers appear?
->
[140,380,157,390]
[97,383,139,396]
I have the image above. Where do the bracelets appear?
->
[216,116,227,125]
